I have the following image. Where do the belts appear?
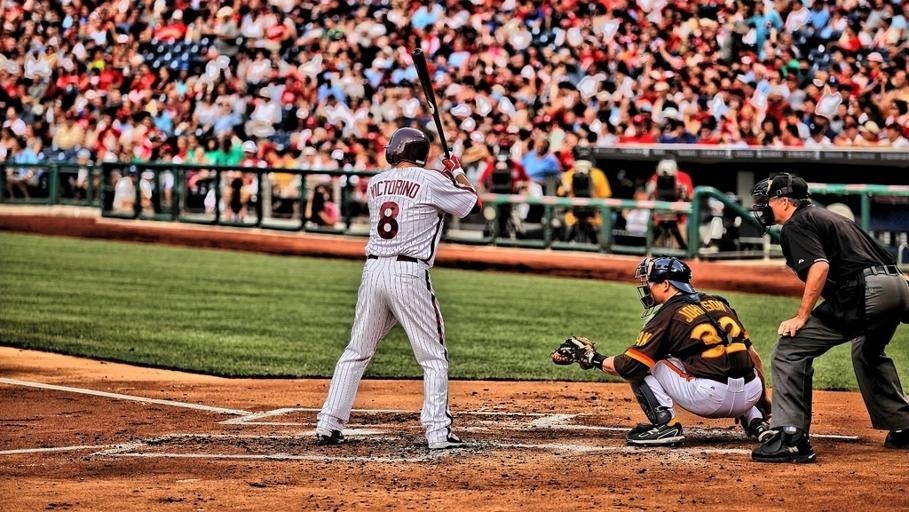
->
[368,254,417,263]
[864,266,902,276]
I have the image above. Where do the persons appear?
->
[549,257,773,448]
[316,125,483,448]
[0,2,909,248]
[746,169,909,464]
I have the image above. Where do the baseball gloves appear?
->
[551,334,596,369]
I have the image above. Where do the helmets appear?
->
[383,127,430,165]
[634,256,698,318]
[750,172,810,238]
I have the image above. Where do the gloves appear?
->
[439,151,465,179]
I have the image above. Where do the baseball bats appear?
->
[411,48,450,158]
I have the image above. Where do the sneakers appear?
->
[884,430,909,449]
[447,433,467,447]
[751,428,816,463]
[747,418,774,442]
[626,422,685,444]
[316,428,344,443]
